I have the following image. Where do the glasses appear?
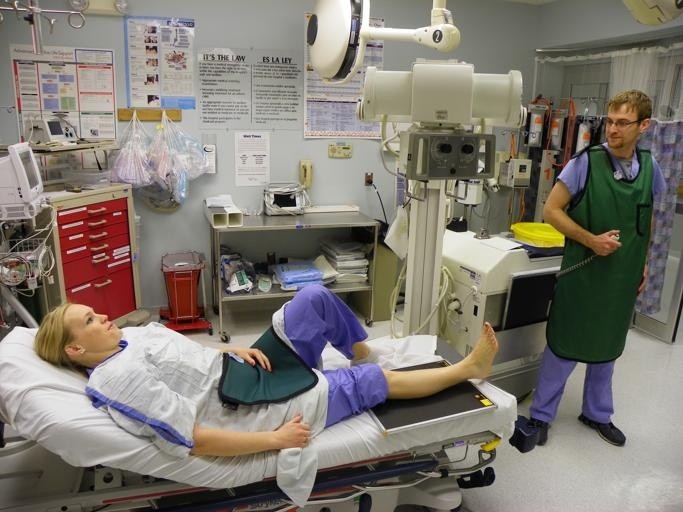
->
[603,116,641,131]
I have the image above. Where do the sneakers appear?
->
[528,414,549,447]
[578,410,626,447]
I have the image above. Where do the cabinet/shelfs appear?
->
[34,184,142,330]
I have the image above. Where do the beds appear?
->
[1,326,518,512]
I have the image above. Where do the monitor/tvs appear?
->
[0,140,45,205]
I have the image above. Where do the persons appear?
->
[530,88,667,448]
[33,285,500,458]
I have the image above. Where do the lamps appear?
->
[64,0,128,17]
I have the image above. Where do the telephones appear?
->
[299,159,313,190]
